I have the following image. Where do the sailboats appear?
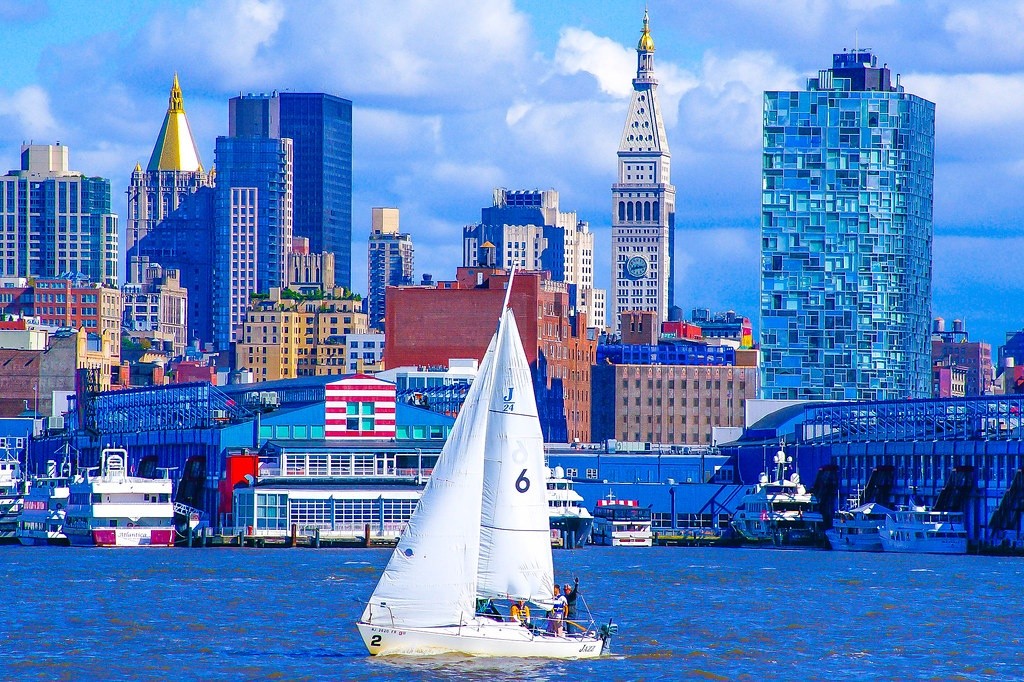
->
[355,262,619,662]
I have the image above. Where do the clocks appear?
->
[626,255,649,277]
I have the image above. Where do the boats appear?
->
[547,466,653,547]
[728,428,970,554]
[0,428,178,548]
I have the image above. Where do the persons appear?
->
[563,577,579,637]
[510,601,537,631]
[476,598,503,622]
[546,584,568,632]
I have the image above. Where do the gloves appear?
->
[575,577,579,583]
